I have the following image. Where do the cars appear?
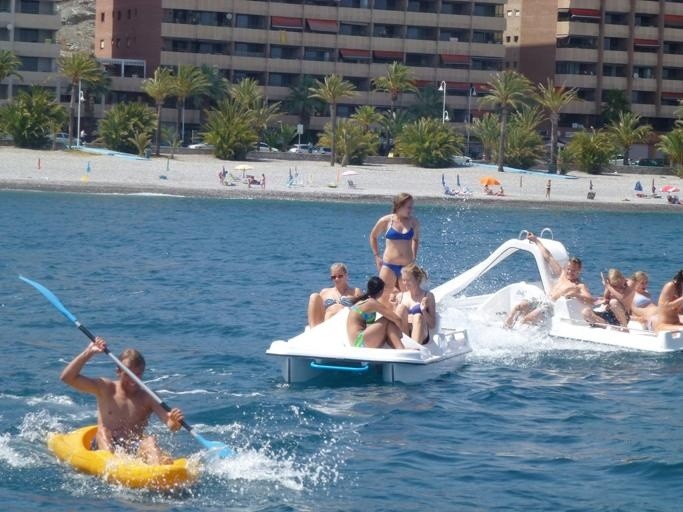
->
[0,128,83,145]
[544,143,565,152]
[187,140,475,169]
[604,151,666,166]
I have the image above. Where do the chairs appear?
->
[347,180,360,190]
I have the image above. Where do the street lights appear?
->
[436,80,450,126]
[463,86,476,152]
[75,90,85,146]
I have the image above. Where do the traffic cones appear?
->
[164,158,171,172]
[35,157,41,171]
[84,160,92,175]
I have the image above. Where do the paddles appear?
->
[19,274,237,458]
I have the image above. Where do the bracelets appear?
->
[373,251,379,257]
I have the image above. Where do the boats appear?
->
[261,306,488,386]
[45,422,201,493]
[430,224,682,355]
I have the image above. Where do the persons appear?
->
[57,335,185,467]
[306,261,363,328]
[260,173,265,189]
[395,262,436,347]
[667,194,680,204]
[344,274,409,348]
[631,270,658,330]
[545,179,551,199]
[483,184,504,196]
[219,171,228,182]
[652,265,682,334]
[247,177,253,186]
[581,268,635,333]
[368,192,421,308]
[505,231,610,333]
